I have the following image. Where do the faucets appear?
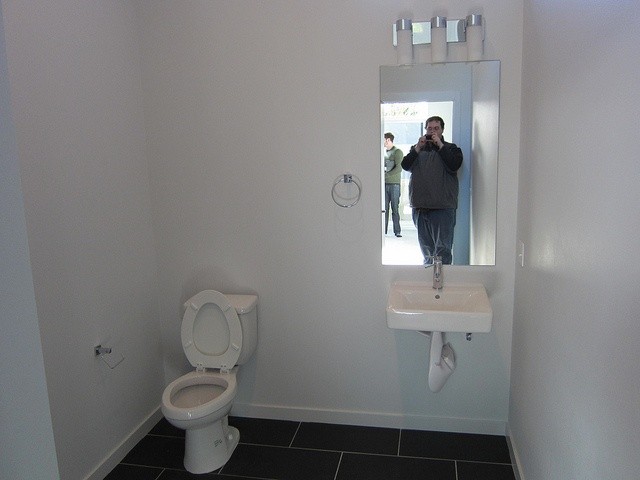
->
[431,256,444,290]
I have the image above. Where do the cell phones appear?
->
[424,134,435,141]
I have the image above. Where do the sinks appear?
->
[387,287,492,334]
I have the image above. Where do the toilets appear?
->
[162,290,258,474]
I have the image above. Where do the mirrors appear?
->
[380,60,499,265]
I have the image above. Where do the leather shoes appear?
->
[395,233,402,237]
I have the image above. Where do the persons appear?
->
[384,132,403,237]
[401,117,463,265]
